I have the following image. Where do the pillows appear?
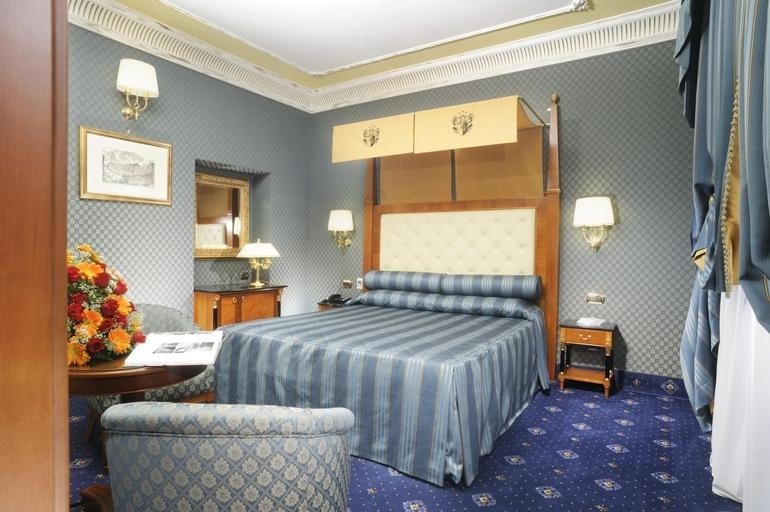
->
[342,270,545,300]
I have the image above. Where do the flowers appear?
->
[67,243,146,366]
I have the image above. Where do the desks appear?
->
[194,282,288,332]
[69,333,208,512]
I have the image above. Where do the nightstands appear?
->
[558,319,617,399]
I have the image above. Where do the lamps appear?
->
[236,237,281,287]
[573,195,614,252]
[327,209,354,249]
[117,57,159,121]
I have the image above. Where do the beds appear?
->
[212,93,561,489]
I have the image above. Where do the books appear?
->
[124,327,224,366]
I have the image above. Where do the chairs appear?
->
[85,301,217,456]
[100,403,356,511]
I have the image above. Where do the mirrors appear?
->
[194,171,251,260]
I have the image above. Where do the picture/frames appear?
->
[78,125,173,206]
